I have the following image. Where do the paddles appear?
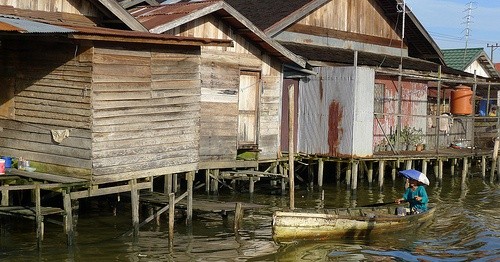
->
[359,197,414,207]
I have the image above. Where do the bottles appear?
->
[18,156,22,169]
[25,161,29,168]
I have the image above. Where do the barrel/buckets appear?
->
[2,155,12,167]
[453,85,474,114]
[0,159,6,175]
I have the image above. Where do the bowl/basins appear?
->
[26,167,36,172]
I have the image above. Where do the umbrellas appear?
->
[399,169,429,185]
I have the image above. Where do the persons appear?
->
[394,178,429,217]
[489,106,497,143]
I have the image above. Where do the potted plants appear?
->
[375,126,426,153]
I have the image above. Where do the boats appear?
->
[271,199,437,246]
